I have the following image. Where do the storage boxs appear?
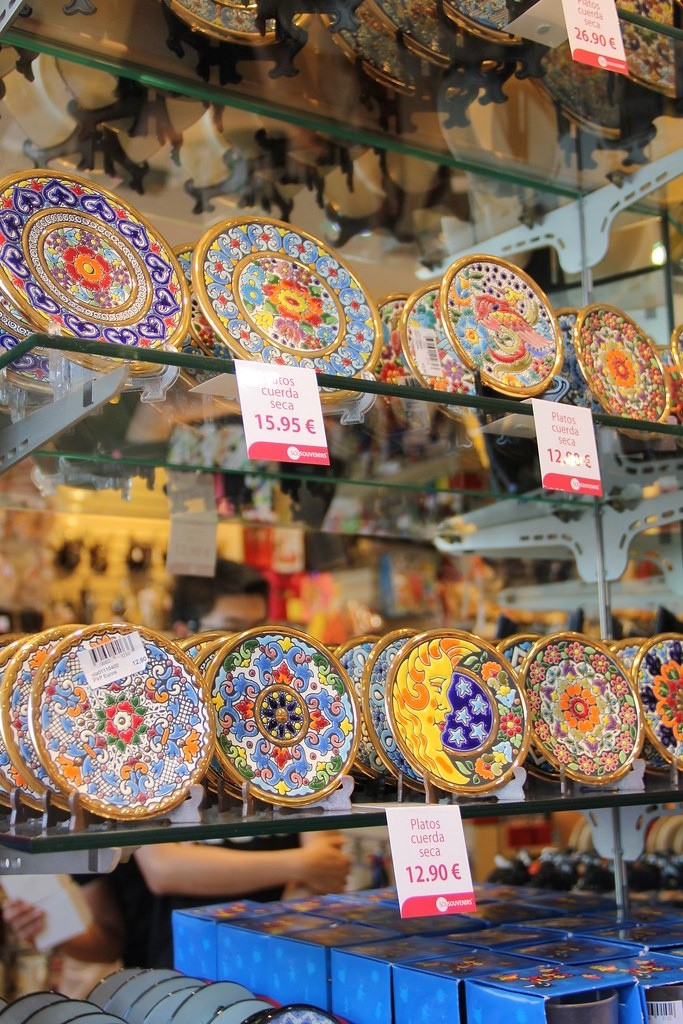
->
[166,889,683,1024]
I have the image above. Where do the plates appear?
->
[0,0,683,1024]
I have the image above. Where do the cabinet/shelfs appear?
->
[0,0,683,853]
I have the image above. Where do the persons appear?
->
[0,559,354,969]
